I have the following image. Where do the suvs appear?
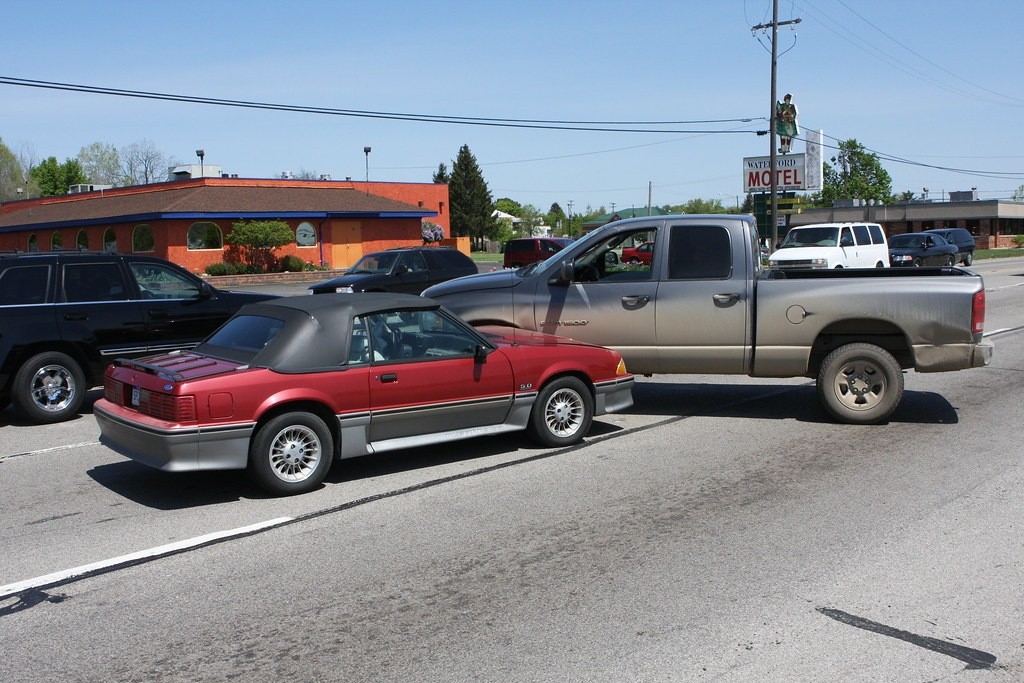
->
[0,248,287,424]
[307,245,479,325]
[766,220,891,270]
[620,241,655,265]
[417,214,995,425]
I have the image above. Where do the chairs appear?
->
[350,335,385,362]
[387,332,402,356]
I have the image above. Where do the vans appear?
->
[502,235,577,269]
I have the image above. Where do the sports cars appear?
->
[94,289,636,497]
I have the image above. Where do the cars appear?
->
[922,227,976,266]
[887,233,962,268]
